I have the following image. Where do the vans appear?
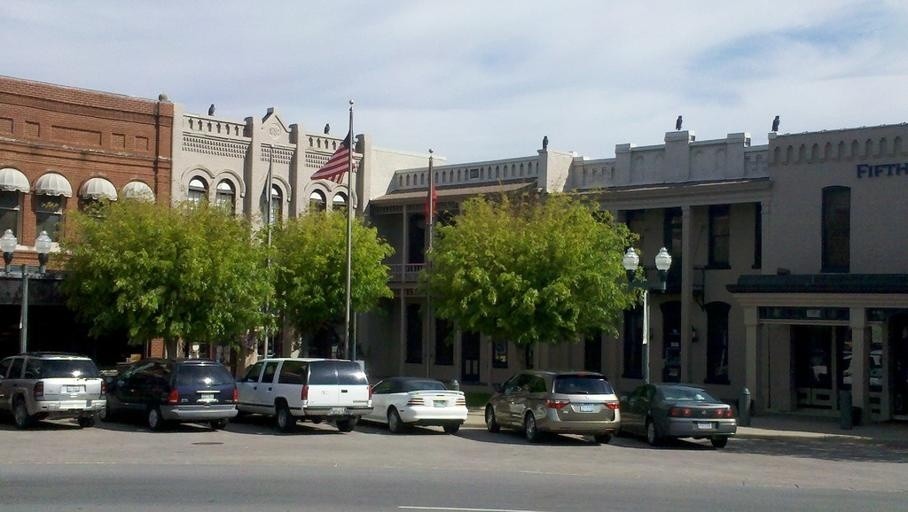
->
[97,357,240,430]
[235,357,374,432]
[484,369,621,444]
[0,352,108,428]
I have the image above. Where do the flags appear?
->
[259,164,272,204]
[423,164,437,224]
[310,116,356,184]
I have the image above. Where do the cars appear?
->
[612,383,738,449]
[355,376,468,434]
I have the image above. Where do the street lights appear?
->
[622,246,672,385]
[0,228,52,354]
